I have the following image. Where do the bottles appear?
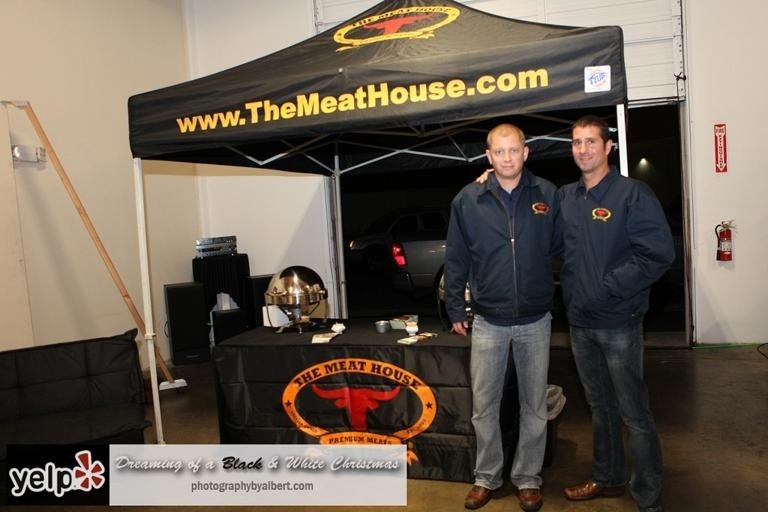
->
[406,322,419,335]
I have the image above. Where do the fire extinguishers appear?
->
[715,219,736,261]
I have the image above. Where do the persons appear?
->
[444,122,564,511]
[475,115,677,511]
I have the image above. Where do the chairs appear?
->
[3,329,148,500]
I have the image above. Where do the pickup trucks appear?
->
[390,228,562,328]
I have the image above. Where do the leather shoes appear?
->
[464,486,491,509]
[565,480,625,499]
[518,489,542,512]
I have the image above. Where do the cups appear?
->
[375,320,390,334]
